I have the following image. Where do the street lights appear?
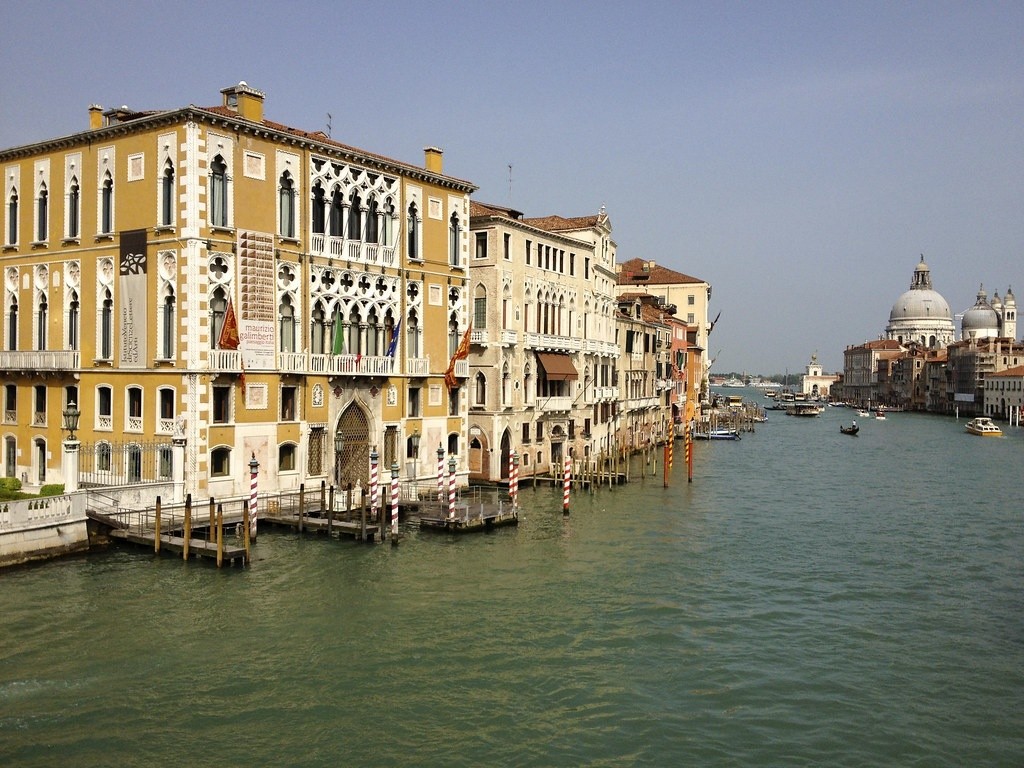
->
[61,400,82,494]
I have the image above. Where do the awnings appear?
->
[537,352,579,381]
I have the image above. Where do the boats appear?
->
[840,425,859,434]
[764,389,847,416]
[873,409,885,420]
[856,409,870,417]
[710,428,736,439]
[964,417,1002,437]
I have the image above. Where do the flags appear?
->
[445,323,472,393]
[386,321,400,357]
[219,297,240,351]
[333,312,346,355]
